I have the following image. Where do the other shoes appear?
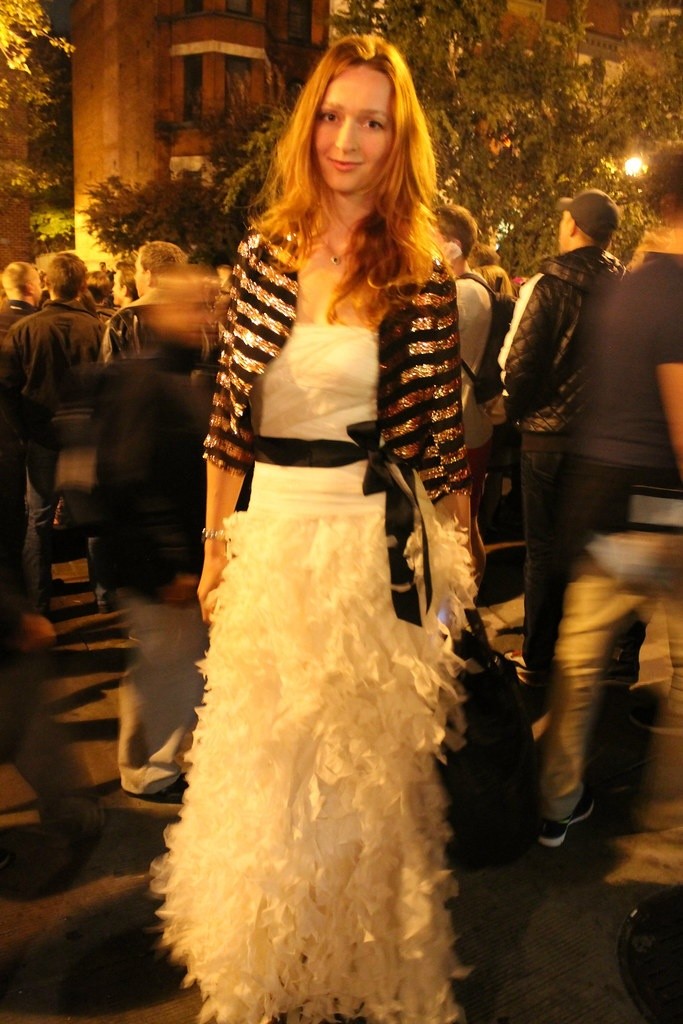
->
[627,702,683,735]
[158,772,188,796]
[97,592,117,612]
[501,649,548,686]
[597,653,640,686]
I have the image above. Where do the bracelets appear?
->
[201,527,226,543]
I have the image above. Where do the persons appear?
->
[145,32,480,1024]
[432,185,683,738]
[0,240,233,882]
[533,143,683,848]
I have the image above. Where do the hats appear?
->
[556,191,618,241]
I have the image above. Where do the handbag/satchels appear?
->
[444,609,542,870]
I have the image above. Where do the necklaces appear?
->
[313,221,348,265]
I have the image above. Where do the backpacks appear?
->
[454,274,515,404]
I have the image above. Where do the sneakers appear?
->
[539,797,595,847]
